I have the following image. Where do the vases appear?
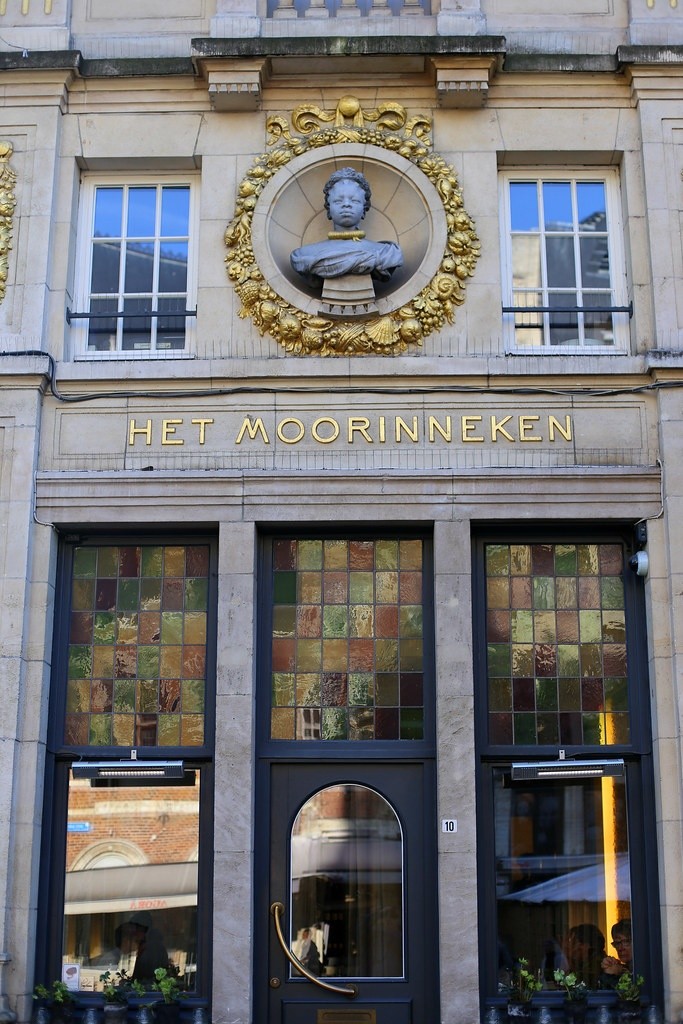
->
[508,1000,532,1024]
[564,999,587,1024]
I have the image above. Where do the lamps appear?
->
[511,757,625,781]
[72,760,184,779]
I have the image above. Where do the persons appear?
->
[567,917,634,990]
[539,942,570,982]
[96,911,171,982]
[289,169,404,284]
[293,902,401,977]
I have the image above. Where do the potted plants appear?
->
[99,970,145,1024]
[611,972,644,1024]
[32,981,74,1024]
[151,968,180,1024]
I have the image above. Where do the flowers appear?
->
[553,968,590,1001]
[498,957,544,1001]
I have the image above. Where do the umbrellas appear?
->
[502,853,633,903]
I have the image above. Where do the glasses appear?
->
[611,940,632,947]
